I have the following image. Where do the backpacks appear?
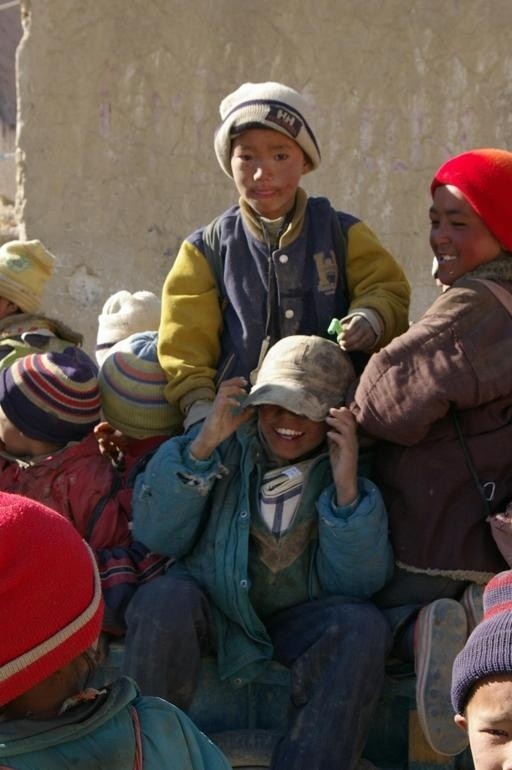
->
[0,337,76,371]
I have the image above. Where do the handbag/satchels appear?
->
[485,503,512,569]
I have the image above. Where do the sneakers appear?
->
[458,579,488,637]
[412,596,470,758]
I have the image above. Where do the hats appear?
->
[213,81,322,179]
[0,239,56,314]
[0,346,103,444]
[246,334,357,424]
[449,569,512,714]
[98,331,185,440]
[430,147,512,253]
[0,491,107,708]
[96,289,162,346]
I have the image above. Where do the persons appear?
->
[1,349,139,565]
[354,149,512,759]
[67,330,183,635]
[446,566,511,770]
[128,335,392,766]
[157,81,411,428]
[95,287,160,371]
[0,237,74,360]
[0,490,233,769]
